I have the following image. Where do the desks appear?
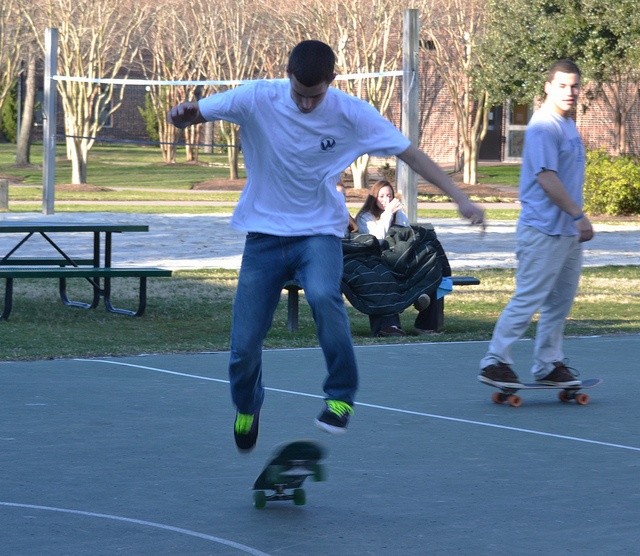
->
[0,218,150,296]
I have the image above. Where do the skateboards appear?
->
[479,378,601,407]
[252,441,327,507]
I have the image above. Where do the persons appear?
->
[355,180,442,335]
[167,40,484,453]
[329,181,359,234]
[477,60,593,386]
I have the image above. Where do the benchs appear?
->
[287,222,479,331]
[0,267,174,316]
[0,257,100,308]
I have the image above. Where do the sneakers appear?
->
[315,400,355,435]
[536,358,582,386]
[379,327,406,336]
[234,408,259,454]
[414,294,430,311]
[478,362,524,388]
[414,327,440,336]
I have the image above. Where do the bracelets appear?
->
[572,213,584,220]
